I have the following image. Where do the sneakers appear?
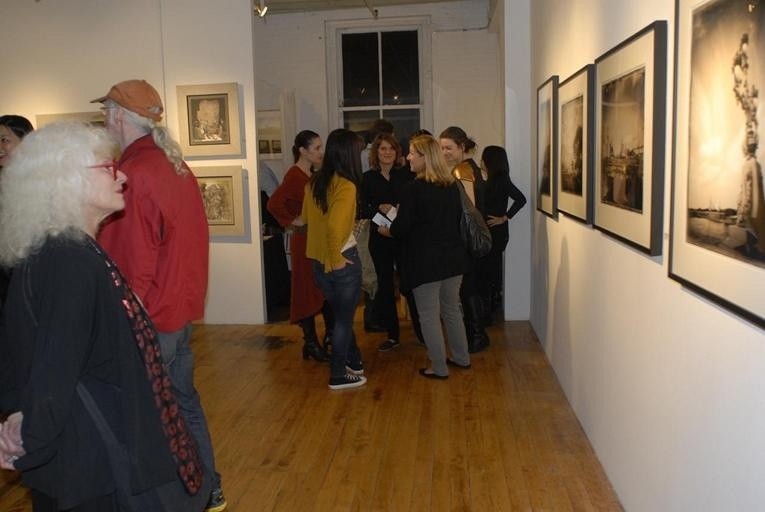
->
[303,322,400,391]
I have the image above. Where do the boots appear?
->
[205,472,228,512]
[467,327,489,352]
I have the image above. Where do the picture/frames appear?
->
[535,75,559,220]
[186,165,246,239]
[176,83,244,159]
[553,62,590,225]
[35,111,104,128]
[591,19,666,256]
[666,1,764,328]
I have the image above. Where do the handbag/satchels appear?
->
[456,180,493,259]
[107,441,211,512]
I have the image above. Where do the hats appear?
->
[90,80,163,122]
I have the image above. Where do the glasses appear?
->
[87,158,119,181]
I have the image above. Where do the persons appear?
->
[91,78,231,512]
[2,119,213,511]
[2,115,35,167]
[259,119,527,389]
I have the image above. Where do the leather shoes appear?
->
[446,358,471,369]
[420,367,449,379]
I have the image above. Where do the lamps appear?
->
[253,0,268,18]
